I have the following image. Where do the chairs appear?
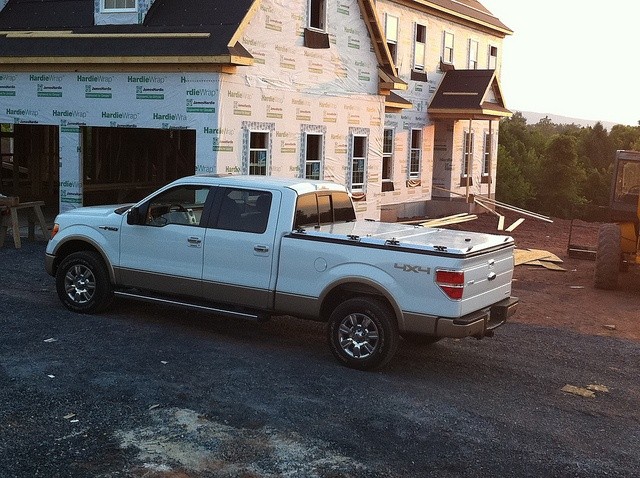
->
[622,163,640,197]
[218,195,237,231]
[242,195,269,233]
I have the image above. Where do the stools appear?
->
[0,201,50,249]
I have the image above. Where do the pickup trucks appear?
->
[43,172,521,371]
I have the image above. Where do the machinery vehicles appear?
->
[593,149,640,292]
